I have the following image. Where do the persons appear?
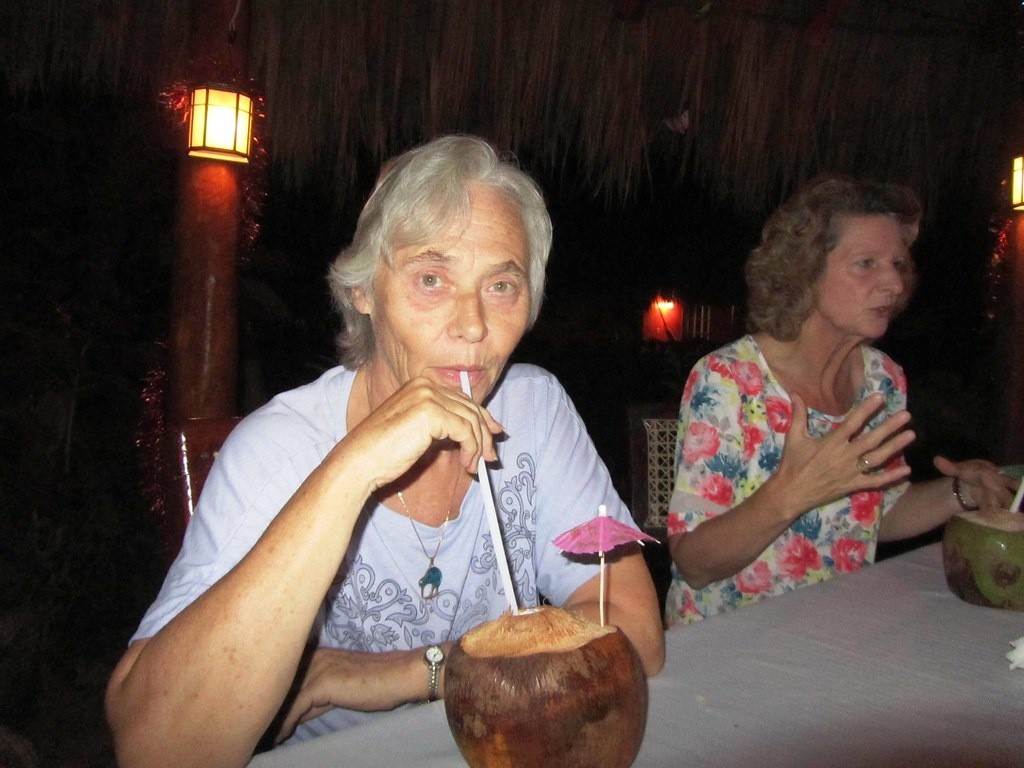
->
[666,177,1024,628]
[105,136,665,768]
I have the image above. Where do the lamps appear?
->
[187,77,255,167]
[1010,154,1024,213]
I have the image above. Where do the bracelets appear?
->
[953,477,978,510]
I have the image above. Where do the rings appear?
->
[856,455,872,472]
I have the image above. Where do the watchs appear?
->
[421,644,446,702]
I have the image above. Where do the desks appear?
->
[246,541,1024,768]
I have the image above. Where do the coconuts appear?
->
[942,506,1024,612]
[443,605,649,768]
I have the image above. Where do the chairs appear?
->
[627,402,680,545]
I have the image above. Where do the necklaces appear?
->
[366,368,461,599]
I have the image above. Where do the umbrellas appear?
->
[552,505,661,626]
